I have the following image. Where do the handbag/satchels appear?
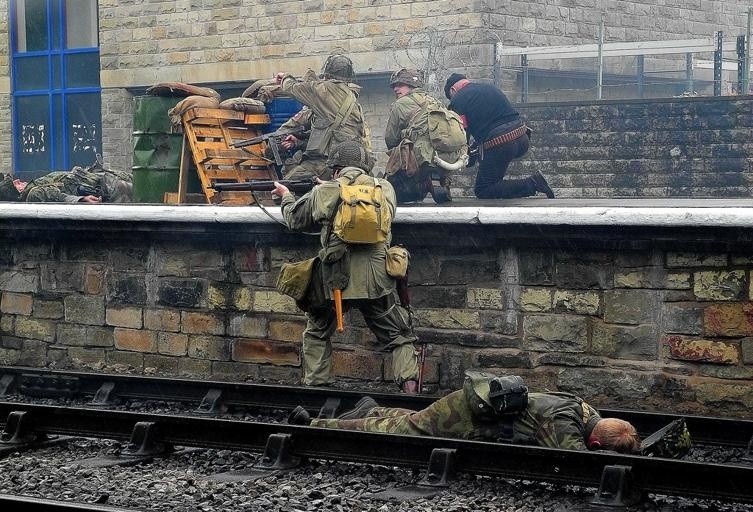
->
[305,117,336,156]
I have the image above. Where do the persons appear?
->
[285,383,641,457]
[270,141,422,395]
[0,163,134,204]
[270,54,373,205]
[443,75,554,199]
[380,67,465,205]
[271,80,376,177]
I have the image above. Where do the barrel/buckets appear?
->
[131,95,203,203]
[262,96,305,134]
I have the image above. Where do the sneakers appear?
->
[528,167,555,199]
[335,395,380,418]
[422,171,448,202]
[288,402,313,425]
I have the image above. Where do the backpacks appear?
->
[331,181,394,248]
[423,104,469,154]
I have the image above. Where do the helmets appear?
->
[324,140,371,175]
[319,54,355,81]
[637,419,693,464]
[388,67,424,90]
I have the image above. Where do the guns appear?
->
[86,153,104,172]
[228,125,310,166]
[207,178,320,198]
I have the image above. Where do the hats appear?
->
[442,73,468,100]
